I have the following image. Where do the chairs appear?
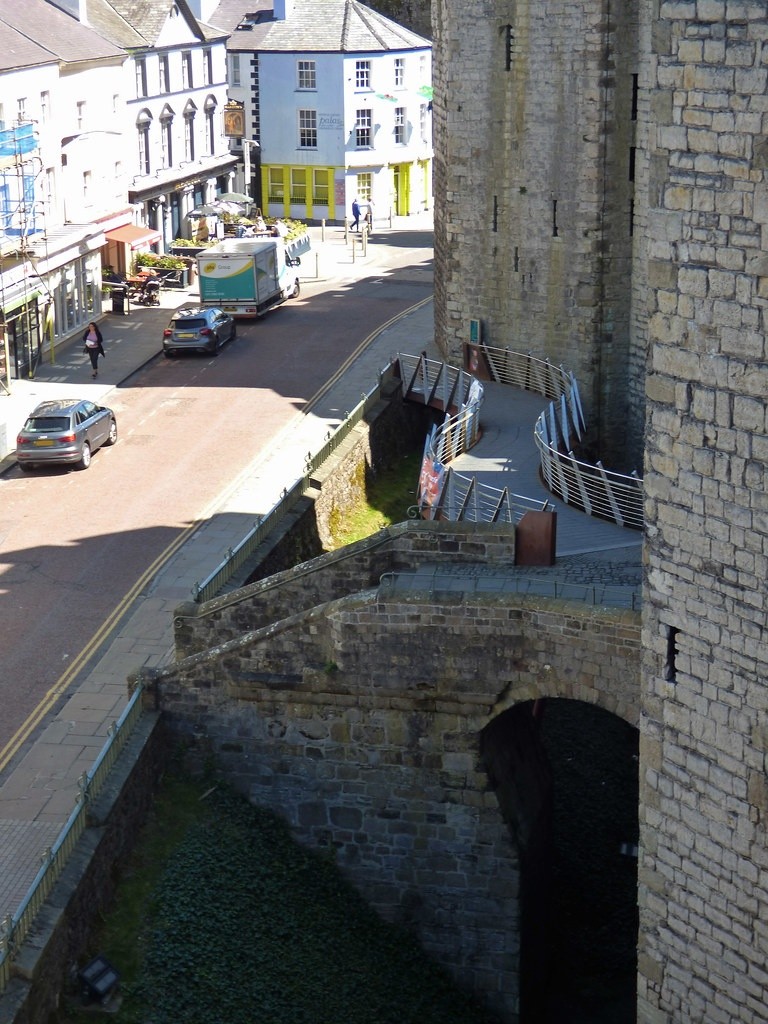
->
[162,271,182,291]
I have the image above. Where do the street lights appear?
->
[243,138,260,219]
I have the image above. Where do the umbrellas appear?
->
[216,193,253,204]
[187,205,227,233]
[208,201,244,214]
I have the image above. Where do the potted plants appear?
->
[171,238,220,258]
[136,250,189,289]
[102,287,110,300]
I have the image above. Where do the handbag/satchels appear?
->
[83,348,88,353]
[364,214,367,221]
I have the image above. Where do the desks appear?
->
[137,272,161,279]
[127,277,147,298]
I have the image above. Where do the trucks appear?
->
[196,236,300,318]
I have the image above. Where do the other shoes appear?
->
[357,231,360,233]
[92,374,96,377]
[96,372,98,374]
[350,225,353,230]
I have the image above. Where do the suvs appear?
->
[16,399,119,473]
[162,304,236,359]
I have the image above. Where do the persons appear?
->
[361,197,373,230]
[108,265,130,296]
[350,198,361,232]
[83,322,105,378]
[141,270,160,294]
[254,217,267,237]
[272,218,288,238]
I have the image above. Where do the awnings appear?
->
[105,225,162,250]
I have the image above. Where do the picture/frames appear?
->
[470,318,481,345]
